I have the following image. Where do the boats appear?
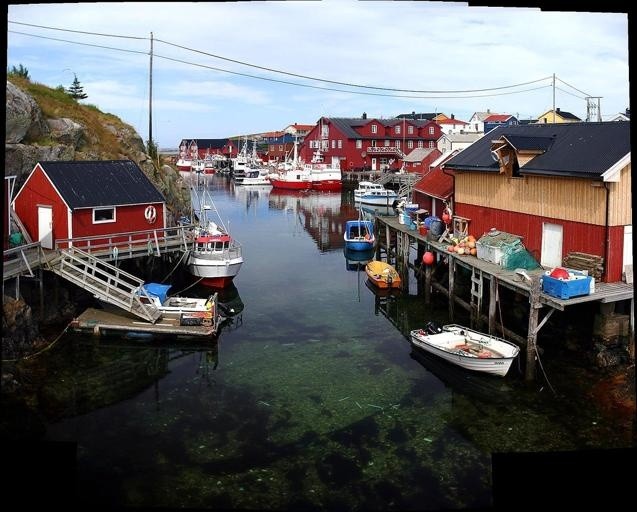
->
[176,134,344,190]
[354,181,397,206]
[410,324,521,378]
[366,261,401,289]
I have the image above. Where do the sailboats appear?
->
[344,185,376,251]
[178,178,244,289]
